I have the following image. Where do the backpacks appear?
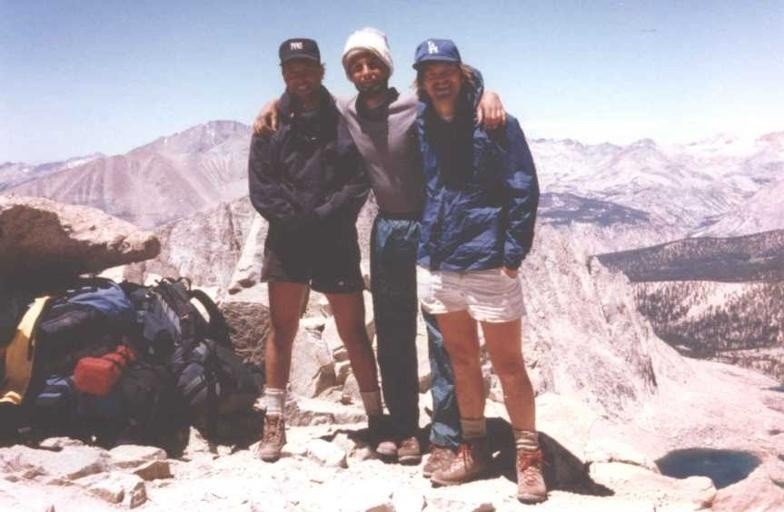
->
[1,278,261,446]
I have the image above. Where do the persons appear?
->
[411,39,548,503]
[253,26,506,478]
[249,37,397,462]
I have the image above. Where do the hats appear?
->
[413,39,461,70]
[342,27,394,81]
[278,39,319,64]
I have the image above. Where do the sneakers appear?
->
[424,443,456,477]
[513,447,551,502]
[259,414,286,461]
[431,434,491,485]
[367,415,422,463]
